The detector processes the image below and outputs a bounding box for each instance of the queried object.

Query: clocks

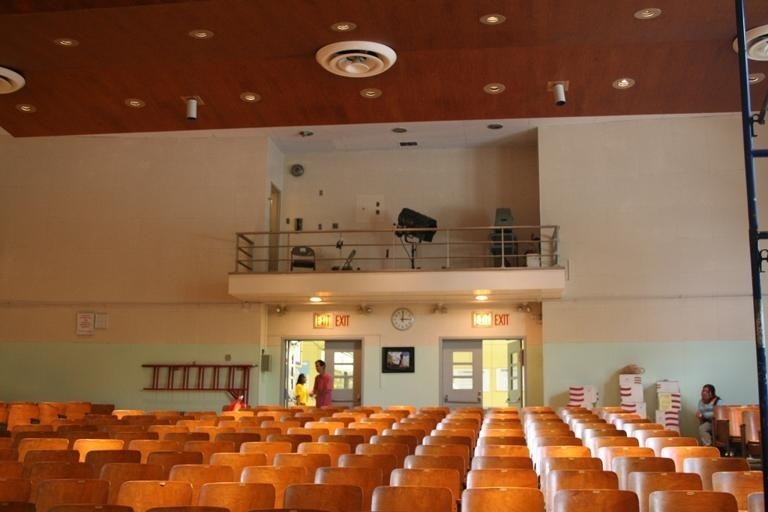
[390,306,415,330]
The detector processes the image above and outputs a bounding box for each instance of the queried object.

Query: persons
[696,384,726,456]
[293,374,307,406]
[309,360,333,408]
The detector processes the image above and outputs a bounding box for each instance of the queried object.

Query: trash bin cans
[527,253,540,267]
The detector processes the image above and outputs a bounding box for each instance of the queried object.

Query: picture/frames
[381,346,415,372]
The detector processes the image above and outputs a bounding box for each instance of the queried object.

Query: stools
[523,251,541,268]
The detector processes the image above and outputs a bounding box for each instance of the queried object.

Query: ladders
[142,364,258,390]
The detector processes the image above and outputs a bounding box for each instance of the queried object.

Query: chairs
[1,403,768,510]
[332,249,357,270]
[488,232,519,266]
[290,246,317,272]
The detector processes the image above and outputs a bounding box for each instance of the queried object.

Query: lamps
[733,24,767,65]
[185,99,197,119]
[551,83,566,107]
[313,40,397,79]
[1,66,24,96]
